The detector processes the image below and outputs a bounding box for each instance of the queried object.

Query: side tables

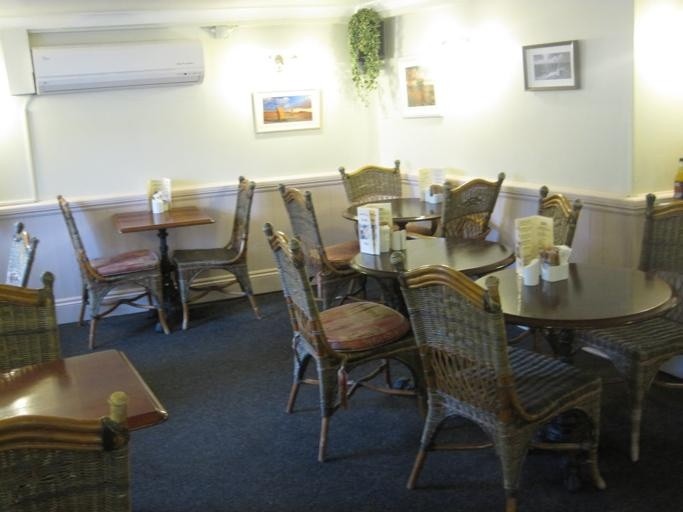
[111,205,214,331]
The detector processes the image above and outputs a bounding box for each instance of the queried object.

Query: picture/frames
[251,90,321,134]
[521,40,582,92]
[398,57,446,119]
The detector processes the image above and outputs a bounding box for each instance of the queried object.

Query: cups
[151,198,163,213]
[378,224,390,254]
[521,256,540,288]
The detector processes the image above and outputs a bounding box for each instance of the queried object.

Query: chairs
[9,223,38,286]
[0,391,129,512]
[57,195,171,350]
[0,272,60,361]
[172,176,262,331]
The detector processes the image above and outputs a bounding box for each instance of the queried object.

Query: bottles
[670,157,682,201]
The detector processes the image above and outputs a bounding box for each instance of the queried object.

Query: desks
[0,349,169,432]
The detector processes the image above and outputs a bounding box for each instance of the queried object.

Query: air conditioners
[30,39,205,96]
